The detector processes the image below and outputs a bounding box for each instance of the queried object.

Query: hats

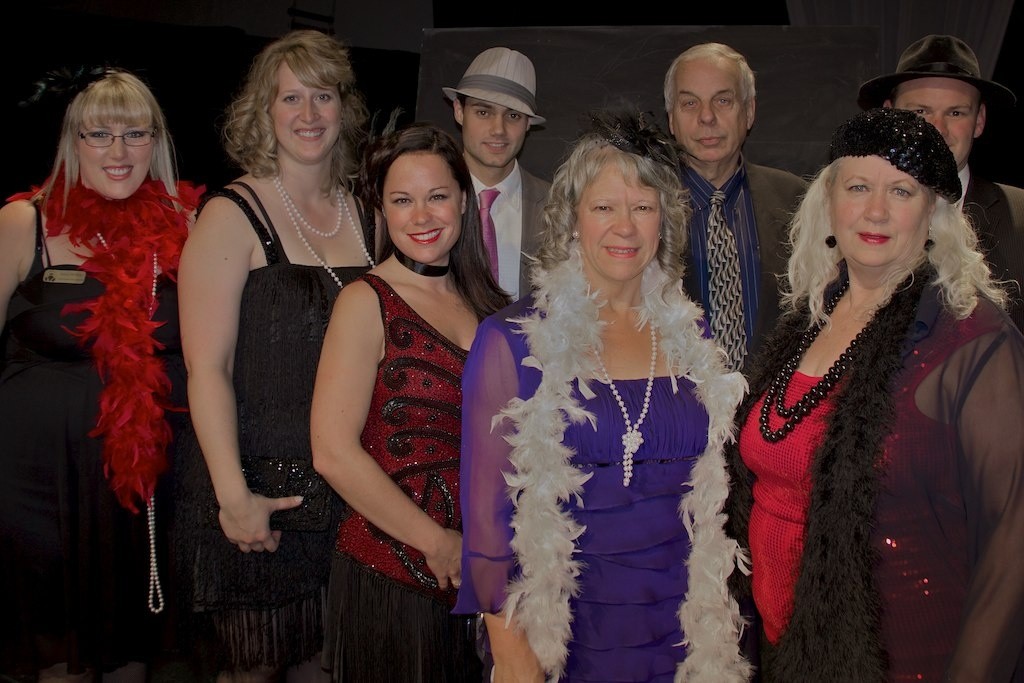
[442,47,548,125]
[856,35,1017,129]
[828,107,963,205]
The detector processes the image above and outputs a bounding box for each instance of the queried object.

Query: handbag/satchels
[207,457,333,534]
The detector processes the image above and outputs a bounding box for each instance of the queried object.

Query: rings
[452,579,463,587]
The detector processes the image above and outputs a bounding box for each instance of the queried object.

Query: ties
[477,189,500,285]
[706,191,749,372]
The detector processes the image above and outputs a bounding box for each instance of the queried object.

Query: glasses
[76,126,165,148]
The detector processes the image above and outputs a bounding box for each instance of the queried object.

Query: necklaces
[759,279,880,444]
[89,230,165,613]
[391,244,452,276]
[274,176,376,289]
[588,314,657,486]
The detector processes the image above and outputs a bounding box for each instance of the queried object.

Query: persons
[451,98,754,683]
[664,42,811,376]
[177,28,384,683]
[859,36,1024,331]
[723,106,1024,683]
[310,106,514,683]
[0,48,206,683]
[442,46,553,304]
